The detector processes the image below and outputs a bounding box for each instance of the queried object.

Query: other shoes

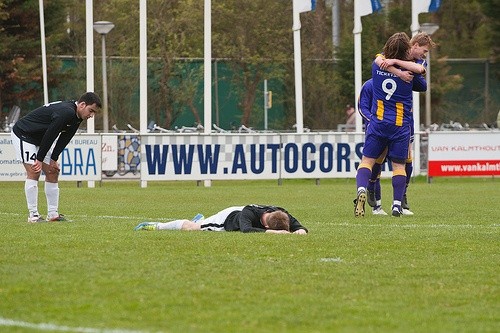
[401,208,414,215]
[353,189,367,218]
[27,215,47,222]
[46,213,68,221]
[134,221,159,231]
[391,200,402,217]
[371,206,388,216]
[191,213,205,222]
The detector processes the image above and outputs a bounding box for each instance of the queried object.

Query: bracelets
[50,159,56,163]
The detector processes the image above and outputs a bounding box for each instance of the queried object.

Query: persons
[353,32,441,218]
[11,92,102,222]
[343,104,356,132]
[134,204,308,236]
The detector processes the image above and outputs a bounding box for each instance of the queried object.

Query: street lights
[420,22,439,130]
[94,20,115,134]
